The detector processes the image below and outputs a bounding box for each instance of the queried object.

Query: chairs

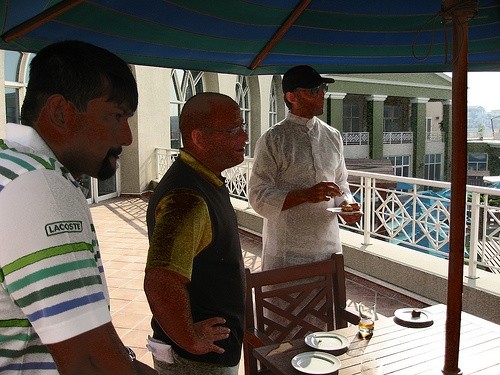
[243,252,361,375]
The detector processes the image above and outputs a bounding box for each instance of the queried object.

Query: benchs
[345,158,397,240]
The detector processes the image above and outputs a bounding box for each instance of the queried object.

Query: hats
[282,65,335,89]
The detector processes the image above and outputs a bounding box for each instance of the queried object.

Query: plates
[327,207,364,215]
[305,331,349,351]
[394,308,435,323]
[291,351,342,375]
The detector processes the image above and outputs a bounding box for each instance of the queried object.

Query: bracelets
[125,346,136,363]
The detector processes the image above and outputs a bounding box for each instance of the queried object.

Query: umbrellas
[0,0,500,375]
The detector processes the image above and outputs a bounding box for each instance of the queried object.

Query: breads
[342,203,361,211]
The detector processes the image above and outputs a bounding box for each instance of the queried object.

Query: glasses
[199,123,245,136]
[294,86,329,95]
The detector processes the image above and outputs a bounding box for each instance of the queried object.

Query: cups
[359,301,376,339]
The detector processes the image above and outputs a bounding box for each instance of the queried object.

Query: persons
[247,64,363,343]
[0,41,159,375]
[144,91,250,375]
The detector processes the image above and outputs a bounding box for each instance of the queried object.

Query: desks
[253,303,500,375]
